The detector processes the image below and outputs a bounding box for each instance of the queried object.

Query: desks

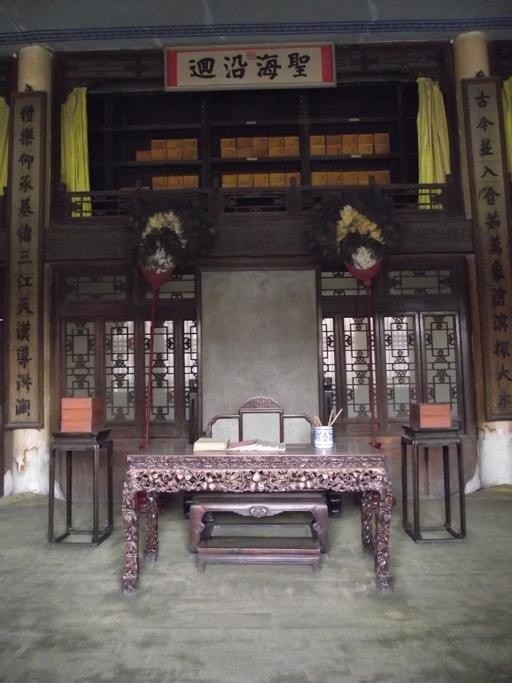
[48,428,114,549]
[123,444,394,595]
[401,425,466,545]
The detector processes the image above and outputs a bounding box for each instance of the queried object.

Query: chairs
[189,396,330,573]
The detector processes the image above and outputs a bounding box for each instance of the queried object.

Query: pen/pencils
[328,408,344,426]
[312,415,322,427]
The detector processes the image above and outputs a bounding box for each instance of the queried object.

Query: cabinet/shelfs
[87,82,416,192]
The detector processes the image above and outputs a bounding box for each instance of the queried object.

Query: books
[192,436,286,451]
